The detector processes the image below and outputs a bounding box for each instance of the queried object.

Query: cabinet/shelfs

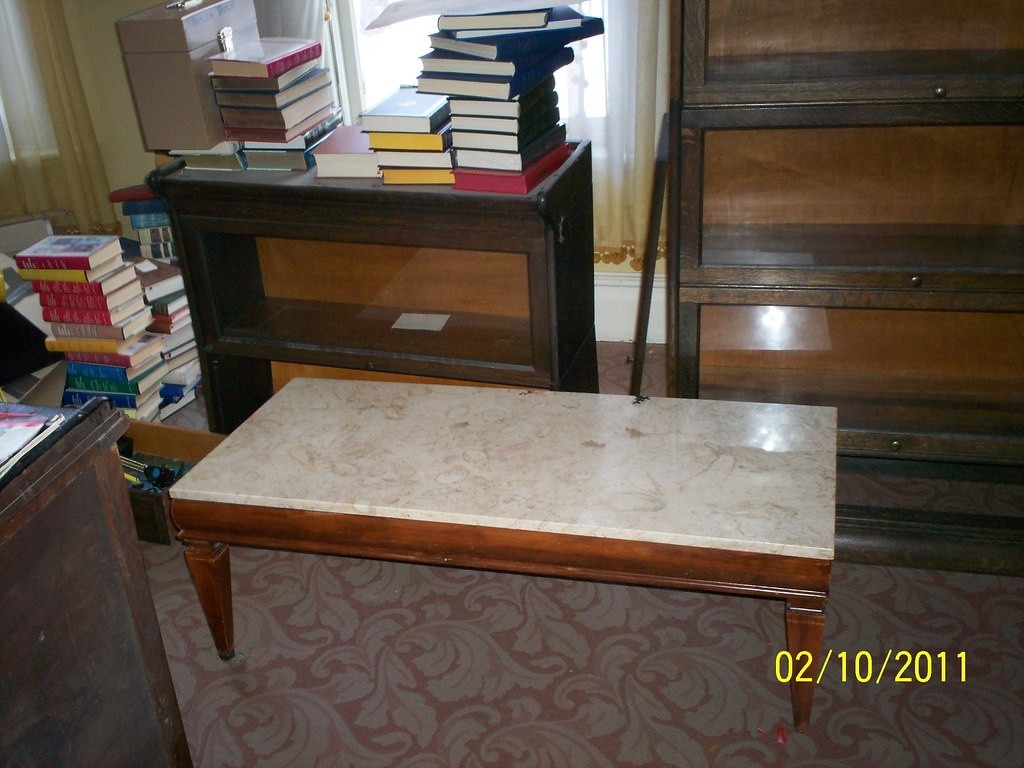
[144,140,602,433]
[664,1,1022,578]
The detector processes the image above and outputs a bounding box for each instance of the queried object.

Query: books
[0,1,607,485]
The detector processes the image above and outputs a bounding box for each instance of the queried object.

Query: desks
[170,375,841,733]
[0,396,197,768]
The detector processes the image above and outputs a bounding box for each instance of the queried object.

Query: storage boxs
[115,0,268,150]
